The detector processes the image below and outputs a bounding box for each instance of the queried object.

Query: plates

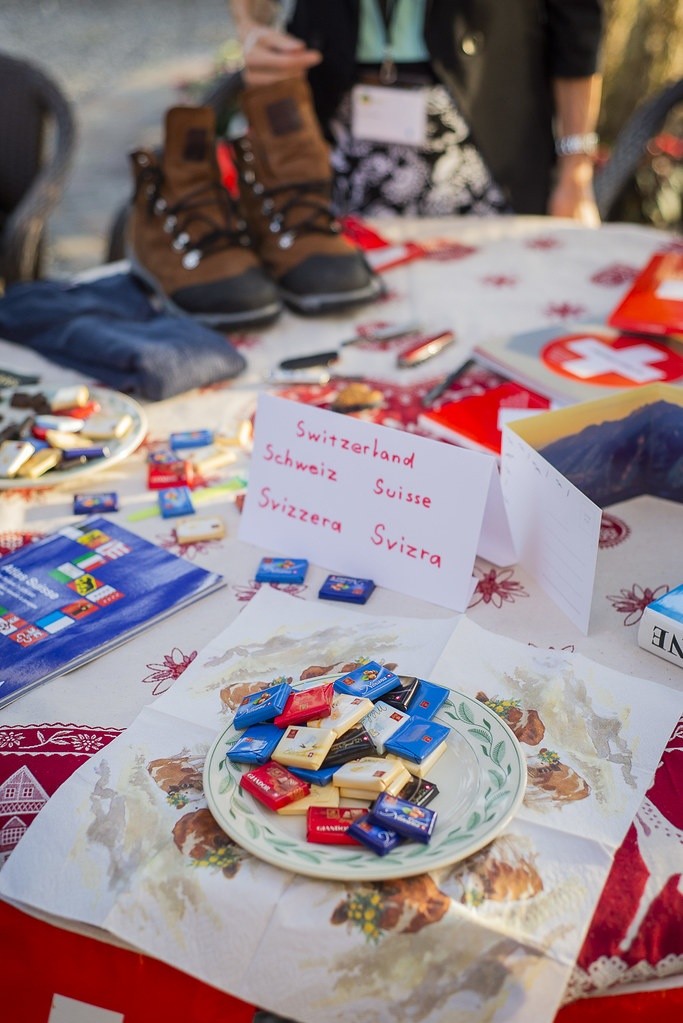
[0,386,147,491]
[205,674,525,881]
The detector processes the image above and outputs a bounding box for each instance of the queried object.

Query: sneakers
[231,77,379,312]
[122,105,282,329]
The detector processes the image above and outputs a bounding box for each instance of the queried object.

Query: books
[609,246,683,340]
[639,584,683,669]
[419,381,563,457]
[0,516,229,710]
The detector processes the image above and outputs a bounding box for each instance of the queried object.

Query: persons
[228,0,612,221]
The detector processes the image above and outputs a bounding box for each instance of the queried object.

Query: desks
[0,213,683,1023]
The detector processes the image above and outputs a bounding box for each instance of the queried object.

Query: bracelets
[556,132,599,156]
[243,30,265,53]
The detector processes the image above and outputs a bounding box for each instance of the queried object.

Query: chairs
[1,55,76,286]
